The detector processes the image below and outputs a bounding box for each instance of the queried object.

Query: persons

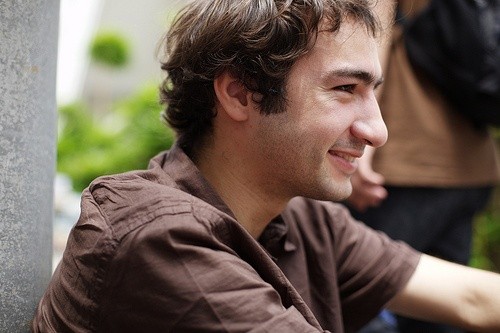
[336,0,500,333]
[26,0,500,333]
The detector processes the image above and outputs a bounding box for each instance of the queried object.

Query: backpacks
[396,0,500,133]
